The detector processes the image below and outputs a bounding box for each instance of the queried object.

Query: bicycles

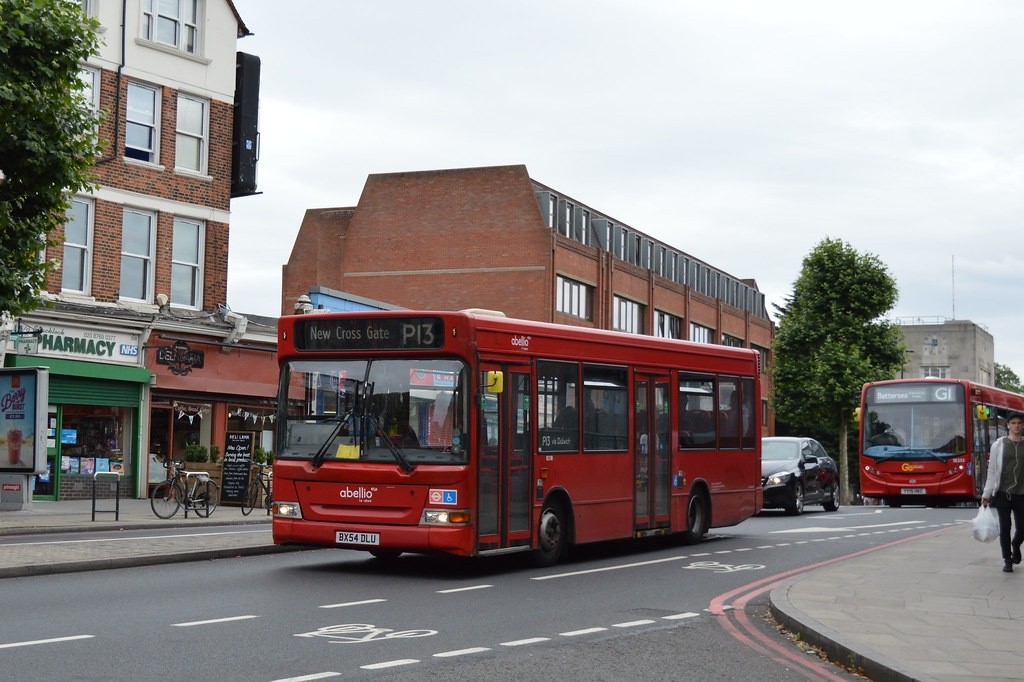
[242,464,272,516]
[151,459,220,519]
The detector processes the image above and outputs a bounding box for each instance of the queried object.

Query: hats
[1006,411,1024,423]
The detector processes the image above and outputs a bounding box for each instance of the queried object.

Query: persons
[488,437,497,447]
[981,412,1024,572]
[729,391,748,437]
[884,417,906,445]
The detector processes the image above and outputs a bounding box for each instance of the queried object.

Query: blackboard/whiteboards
[217,431,256,507]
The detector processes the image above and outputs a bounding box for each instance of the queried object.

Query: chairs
[586,408,731,450]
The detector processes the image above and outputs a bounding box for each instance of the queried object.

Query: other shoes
[1002,559,1013,572]
[1012,544,1022,565]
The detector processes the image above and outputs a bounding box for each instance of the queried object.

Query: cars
[761,436,840,515]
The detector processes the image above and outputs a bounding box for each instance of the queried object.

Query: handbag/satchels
[971,503,1000,543]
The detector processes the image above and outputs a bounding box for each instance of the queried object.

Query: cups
[8,431,23,464]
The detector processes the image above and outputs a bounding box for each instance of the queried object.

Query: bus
[271,309,764,567]
[853,376,1024,508]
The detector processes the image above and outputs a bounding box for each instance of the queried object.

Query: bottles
[393,418,397,433]
